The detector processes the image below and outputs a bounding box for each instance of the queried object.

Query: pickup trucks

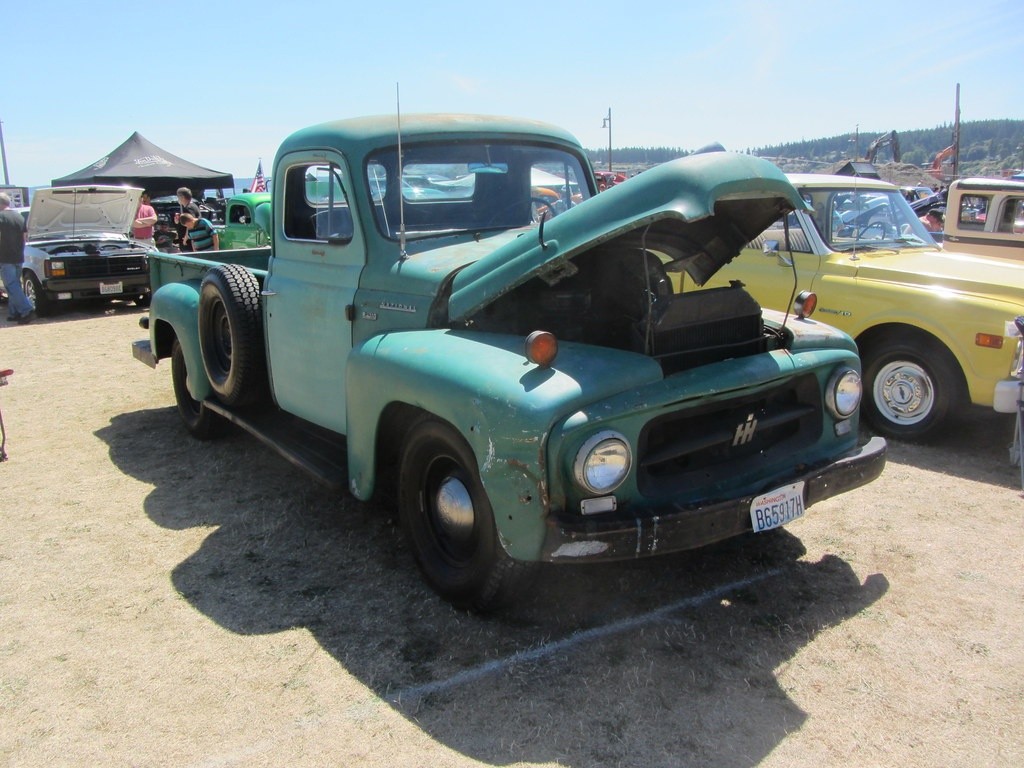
[133,114,886,617]
[937,178,1024,261]
[210,193,271,249]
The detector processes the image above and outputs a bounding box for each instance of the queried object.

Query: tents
[433,162,578,188]
[51,131,235,200]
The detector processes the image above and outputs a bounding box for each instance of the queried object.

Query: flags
[251,161,265,192]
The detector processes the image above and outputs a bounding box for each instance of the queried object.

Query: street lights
[600,107,612,172]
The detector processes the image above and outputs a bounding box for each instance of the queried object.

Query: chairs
[472,171,526,226]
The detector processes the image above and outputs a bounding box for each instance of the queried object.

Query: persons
[916,180,923,187]
[131,195,157,248]
[551,185,577,218]
[931,183,950,202]
[174,187,200,253]
[903,209,944,235]
[0,193,37,325]
[832,201,844,232]
[153,223,182,253]
[180,213,219,252]
[599,175,616,193]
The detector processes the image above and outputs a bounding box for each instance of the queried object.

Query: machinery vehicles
[924,145,955,178]
[865,130,900,164]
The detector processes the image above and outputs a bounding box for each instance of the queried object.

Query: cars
[0,185,160,317]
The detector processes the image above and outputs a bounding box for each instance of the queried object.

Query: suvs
[639,173,1024,440]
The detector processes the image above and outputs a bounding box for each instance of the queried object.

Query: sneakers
[6,310,38,325]
